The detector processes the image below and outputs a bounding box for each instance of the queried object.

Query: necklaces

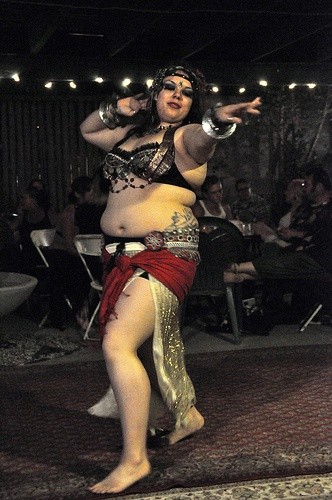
[141,124,174,136]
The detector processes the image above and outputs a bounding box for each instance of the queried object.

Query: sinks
[0,272,39,317]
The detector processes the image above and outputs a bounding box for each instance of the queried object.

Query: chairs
[73,234,104,340]
[183,216,247,345]
[31,230,72,330]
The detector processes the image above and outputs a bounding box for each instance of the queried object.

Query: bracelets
[202,105,237,140]
[99,92,122,129]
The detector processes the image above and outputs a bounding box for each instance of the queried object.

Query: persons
[221,175,332,283]
[45,175,94,332]
[79,62,261,495]
[8,179,55,275]
[277,178,311,230]
[230,180,270,225]
[190,174,233,221]
[75,165,110,232]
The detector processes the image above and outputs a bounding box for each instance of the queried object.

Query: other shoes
[217,312,233,333]
[239,308,272,336]
[279,301,304,324]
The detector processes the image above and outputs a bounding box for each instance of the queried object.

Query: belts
[104,242,198,254]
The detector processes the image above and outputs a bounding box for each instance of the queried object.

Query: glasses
[238,187,249,191]
